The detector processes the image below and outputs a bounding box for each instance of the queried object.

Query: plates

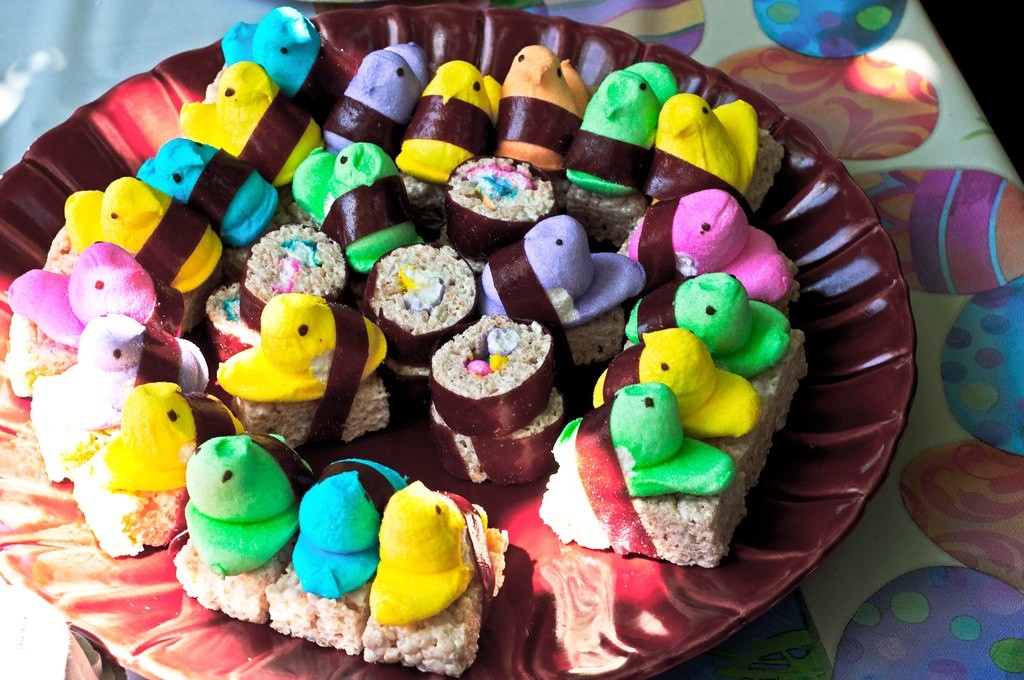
[0,3,918,680]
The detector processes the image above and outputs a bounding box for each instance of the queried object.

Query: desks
[0,0,1024,680]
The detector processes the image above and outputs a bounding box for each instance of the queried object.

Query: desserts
[0,6,810,673]
